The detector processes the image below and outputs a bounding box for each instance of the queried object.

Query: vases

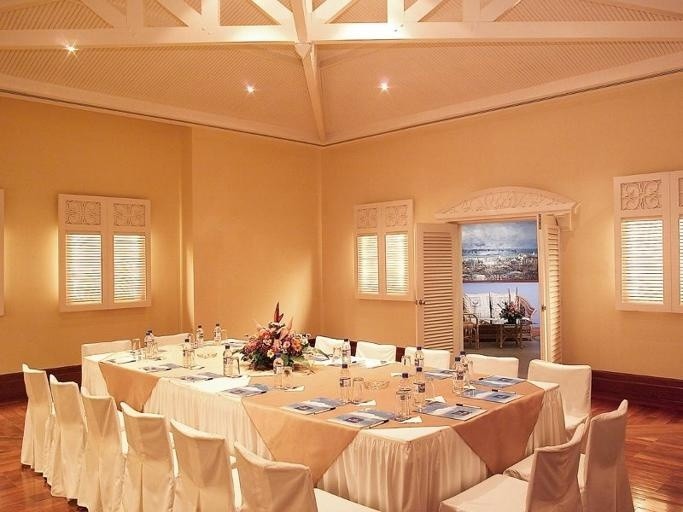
[265,358,295,370]
[507,319,518,325]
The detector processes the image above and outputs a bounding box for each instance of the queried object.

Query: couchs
[463,292,538,342]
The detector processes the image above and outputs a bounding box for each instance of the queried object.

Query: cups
[231,357,240,377]
[331,346,342,367]
[221,329,227,344]
[352,377,366,404]
[282,368,294,392]
[400,355,412,378]
[393,390,412,421]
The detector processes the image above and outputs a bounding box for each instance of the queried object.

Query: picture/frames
[463,247,539,285]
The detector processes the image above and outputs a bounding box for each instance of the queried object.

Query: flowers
[232,302,328,376]
[498,286,526,320]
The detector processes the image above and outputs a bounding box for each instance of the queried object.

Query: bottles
[413,368,425,404]
[273,353,284,388]
[223,345,233,375]
[400,373,413,415]
[144,333,152,359]
[339,364,352,403]
[342,338,351,366]
[414,347,424,368]
[459,351,468,387]
[214,324,222,344]
[148,331,155,358]
[183,339,191,365]
[451,357,464,392]
[196,326,204,347]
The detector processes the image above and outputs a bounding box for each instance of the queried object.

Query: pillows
[463,291,490,318]
[490,291,516,318]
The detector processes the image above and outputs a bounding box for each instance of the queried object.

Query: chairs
[405,346,451,372]
[529,359,592,443]
[154,332,191,347]
[48,374,124,500]
[172,418,243,512]
[77,386,176,512]
[463,313,480,350]
[355,340,397,363]
[120,400,236,512]
[20,363,57,477]
[466,354,519,378]
[315,336,349,355]
[438,421,587,512]
[234,441,383,512]
[502,398,635,512]
[81,340,132,366]
